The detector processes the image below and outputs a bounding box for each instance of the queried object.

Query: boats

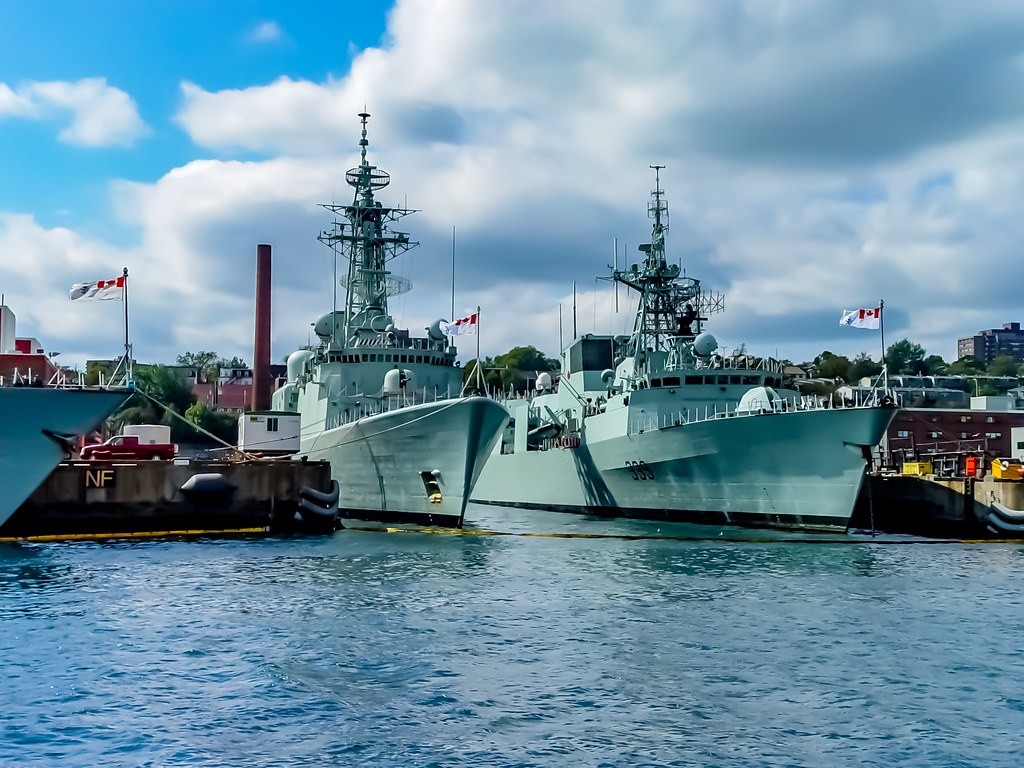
[848,299,1024,540]
[0,266,136,528]
[272,104,512,530]
[0,242,340,541]
[469,162,899,534]
[527,423,563,445]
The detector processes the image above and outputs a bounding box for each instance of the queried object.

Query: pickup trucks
[80,435,176,461]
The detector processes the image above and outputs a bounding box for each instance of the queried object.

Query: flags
[442,313,477,337]
[839,308,880,329]
[68,275,125,301]
